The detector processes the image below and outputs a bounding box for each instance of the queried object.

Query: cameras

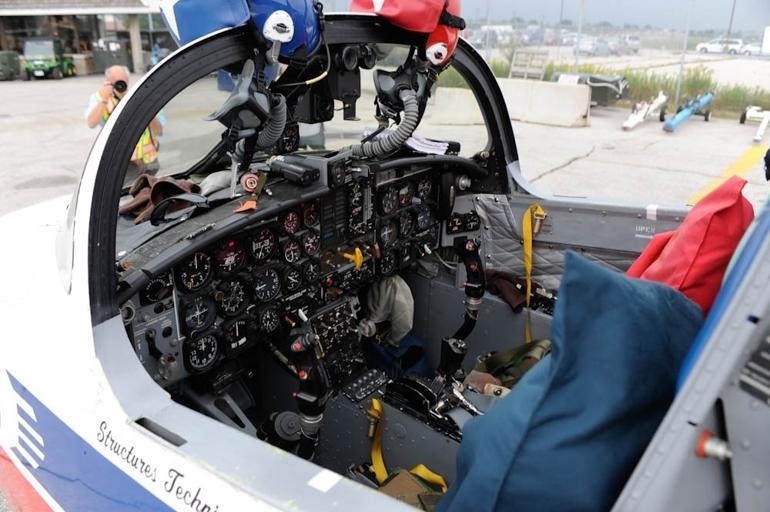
[111,80,127,99]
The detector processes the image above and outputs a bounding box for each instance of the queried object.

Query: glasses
[150,193,210,226]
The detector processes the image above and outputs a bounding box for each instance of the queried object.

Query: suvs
[20,37,76,80]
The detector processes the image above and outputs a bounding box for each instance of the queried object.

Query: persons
[87,65,165,189]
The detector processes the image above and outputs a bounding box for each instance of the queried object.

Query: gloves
[119,174,203,225]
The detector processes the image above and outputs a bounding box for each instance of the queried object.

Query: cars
[695,36,762,57]
[466,25,640,56]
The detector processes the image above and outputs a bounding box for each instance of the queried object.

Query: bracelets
[99,97,108,104]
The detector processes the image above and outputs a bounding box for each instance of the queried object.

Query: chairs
[432,180,769,511]
